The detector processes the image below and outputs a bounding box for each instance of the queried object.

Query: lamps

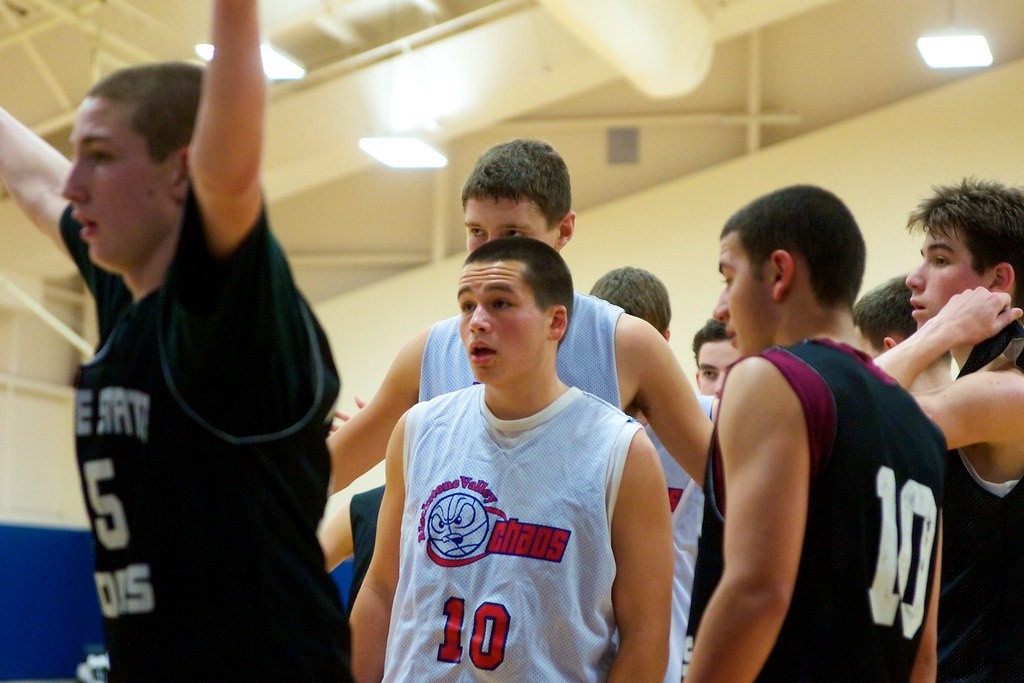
[916,0,994,69]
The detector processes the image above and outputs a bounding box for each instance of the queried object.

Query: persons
[326,139,740,683]
[874,179,1023,683]
[681,184,947,683]
[348,238,673,683]
[855,273,952,395]
[1,1,354,683]
